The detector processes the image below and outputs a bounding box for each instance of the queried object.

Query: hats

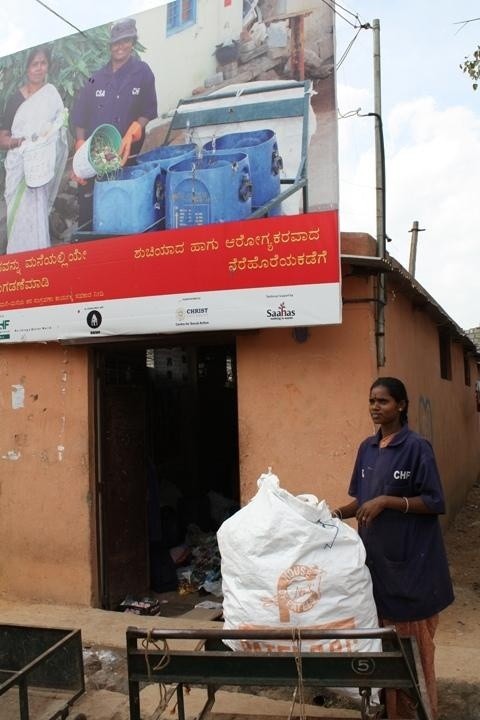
[110,22,137,43]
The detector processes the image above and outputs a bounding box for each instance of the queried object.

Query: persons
[1,47,68,254]
[331,376,456,720]
[73,18,158,230]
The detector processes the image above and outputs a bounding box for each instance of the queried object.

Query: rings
[362,516,366,521]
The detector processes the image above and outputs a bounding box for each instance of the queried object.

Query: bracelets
[331,510,339,517]
[401,497,409,514]
[335,508,342,521]
